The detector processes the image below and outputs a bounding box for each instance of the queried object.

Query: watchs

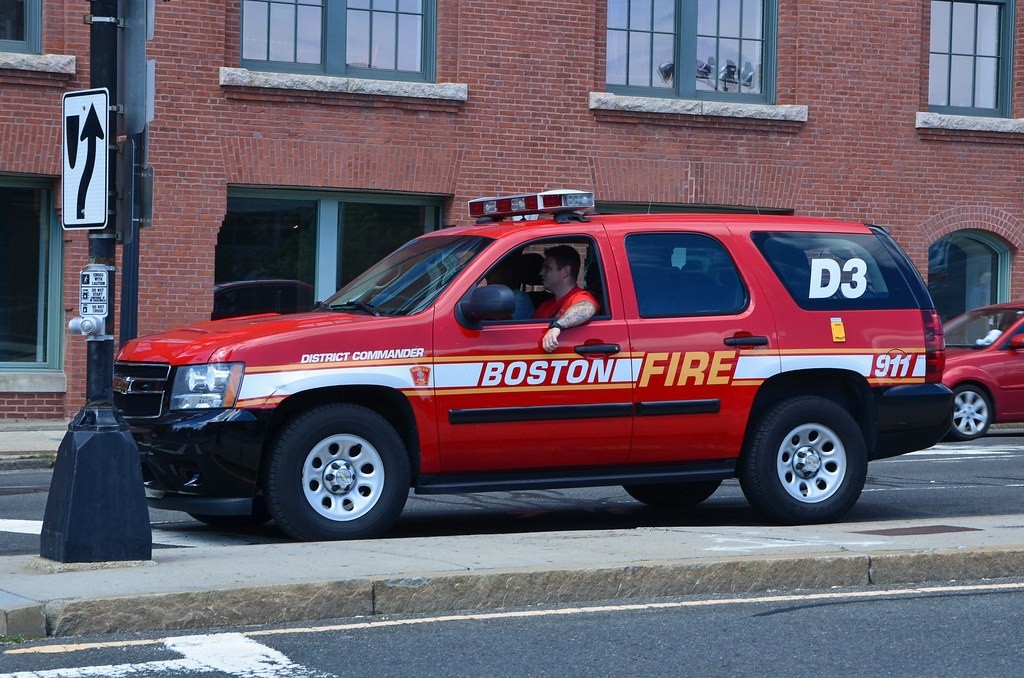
[548,321,562,331]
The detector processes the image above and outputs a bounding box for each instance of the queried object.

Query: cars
[944,301,1024,440]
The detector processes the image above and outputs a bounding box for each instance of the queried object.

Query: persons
[484,245,600,353]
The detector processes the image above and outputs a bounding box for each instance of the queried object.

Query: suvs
[108,186,956,539]
